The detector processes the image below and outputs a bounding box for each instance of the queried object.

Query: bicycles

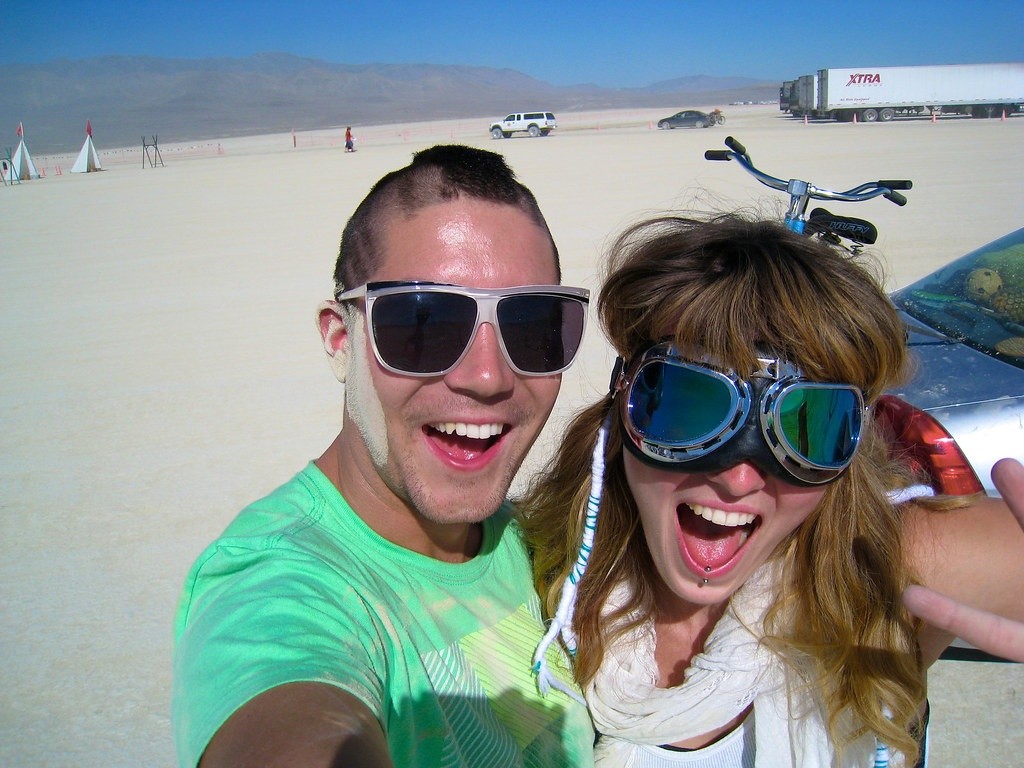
[708,111,726,126]
[710,139,912,258]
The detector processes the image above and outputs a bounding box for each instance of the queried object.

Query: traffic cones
[802,114,808,126]
[1000,109,1007,123]
[853,113,857,124]
[932,113,936,123]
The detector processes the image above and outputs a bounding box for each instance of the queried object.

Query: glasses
[608,343,870,486]
[332,279,590,379]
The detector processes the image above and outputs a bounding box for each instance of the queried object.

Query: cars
[876,231,1024,496]
[657,110,717,130]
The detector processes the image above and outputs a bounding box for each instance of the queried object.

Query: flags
[87,121,92,136]
[16,123,24,137]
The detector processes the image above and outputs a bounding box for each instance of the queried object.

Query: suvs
[488,111,557,140]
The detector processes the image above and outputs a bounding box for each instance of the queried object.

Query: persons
[173,140,596,768]
[517,213,1024,768]
[345,127,353,152]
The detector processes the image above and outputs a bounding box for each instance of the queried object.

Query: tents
[4,140,38,182]
[71,135,102,172]
